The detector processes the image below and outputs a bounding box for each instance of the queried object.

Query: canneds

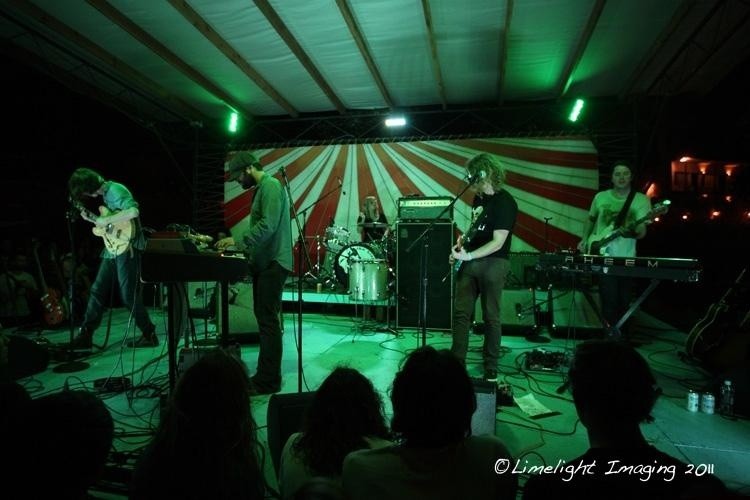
[316,283,323,293]
[687,389,699,412]
[701,392,716,414]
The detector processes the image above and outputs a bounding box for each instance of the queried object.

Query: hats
[227,150,256,182]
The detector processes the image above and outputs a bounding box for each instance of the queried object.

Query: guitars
[32,247,65,326]
[686,268,749,359]
[69,195,135,255]
[578,203,669,254]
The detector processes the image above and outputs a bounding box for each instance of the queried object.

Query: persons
[447,153,518,381]
[57,168,159,348]
[0,250,40,330]
[140,337,728,500]
[574,158,653,336]
[357,196,388,321]
[213,150,295,396]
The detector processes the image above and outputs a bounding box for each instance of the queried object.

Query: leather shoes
[486,368,498,379]
[61,337,88,350]
[128,336,158,347]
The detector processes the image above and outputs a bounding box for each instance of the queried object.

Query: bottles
[719,380,736,421]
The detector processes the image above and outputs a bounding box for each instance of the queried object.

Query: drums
[349,259,389,301]
[324,226,351,249]
[333,241,386,289]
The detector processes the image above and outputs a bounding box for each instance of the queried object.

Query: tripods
[301,238,337,289]
[377,250,403,335]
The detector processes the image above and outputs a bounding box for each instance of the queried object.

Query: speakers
[163,278,218,318]
[217,282,284,345]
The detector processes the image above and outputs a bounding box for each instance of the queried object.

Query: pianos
[552,252,700,270]
[141,235,247,283]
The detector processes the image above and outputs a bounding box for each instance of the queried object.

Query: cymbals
[359,222,387,228]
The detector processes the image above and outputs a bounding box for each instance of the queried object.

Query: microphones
[281,166,288,178]
[470,170,486,184]
[337,178,347,197]
